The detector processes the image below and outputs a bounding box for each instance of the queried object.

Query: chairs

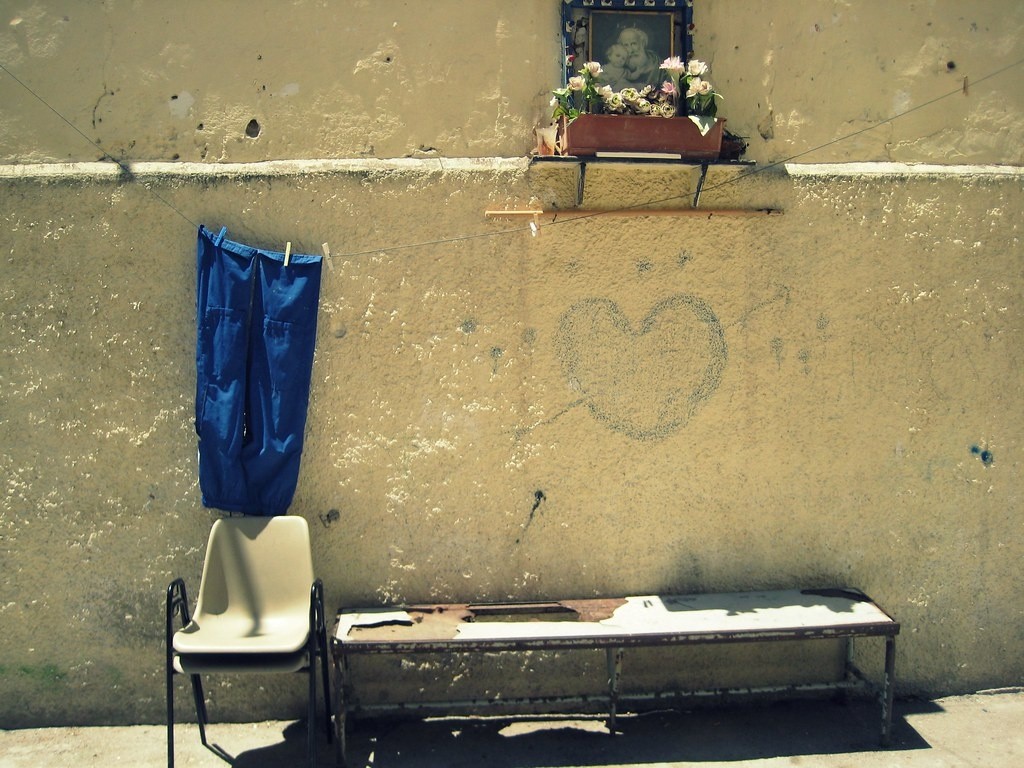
[166,515,332,768]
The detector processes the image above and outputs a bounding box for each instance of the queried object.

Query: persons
[598,27,667,94]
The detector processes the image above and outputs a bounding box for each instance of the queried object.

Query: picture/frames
[562,0,692,110]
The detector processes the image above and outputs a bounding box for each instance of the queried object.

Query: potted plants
[550,56,726,161]
